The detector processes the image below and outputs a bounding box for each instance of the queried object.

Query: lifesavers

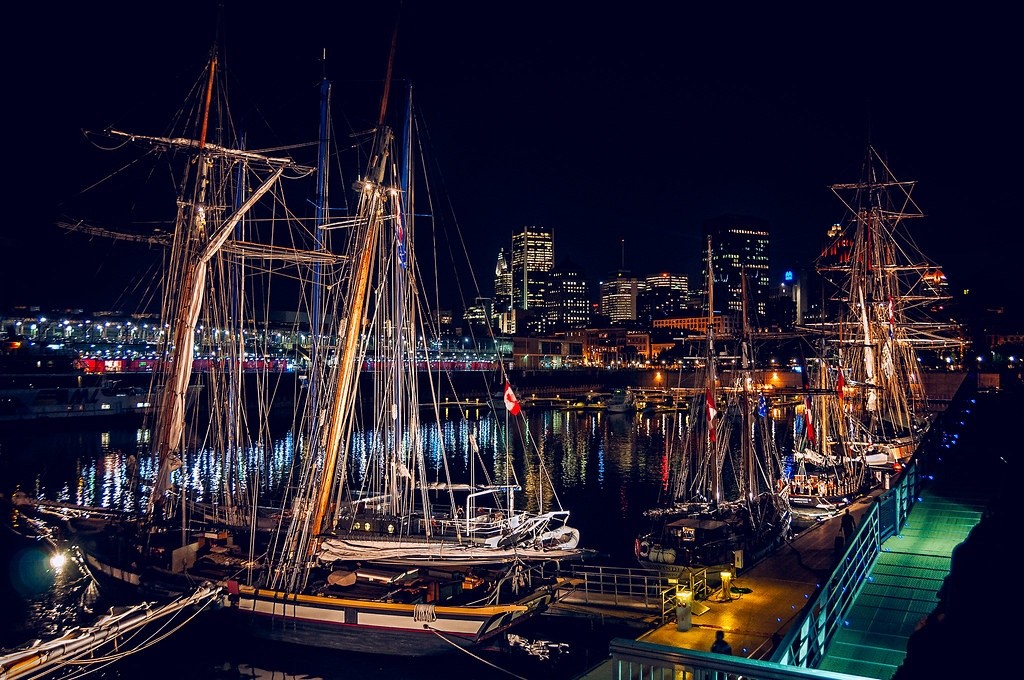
[640,541,650,558]
[634,539,640,555]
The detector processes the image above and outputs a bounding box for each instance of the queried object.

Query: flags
[503,379,521,415]
[805,398,816,446]
[838,365,846,399]
[887,293,894,324]
[753,393,769,422]
[706,387,718,442]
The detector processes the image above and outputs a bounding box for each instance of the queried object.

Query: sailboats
[625,142,973,570]
[6,53,587,655]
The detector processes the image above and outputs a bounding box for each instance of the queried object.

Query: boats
[2,371,204,421]
[558,387,801,414]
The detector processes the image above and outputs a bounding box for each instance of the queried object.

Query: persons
[711,630,732,680]
[377,511,450,537]
[457,505,464,518]
[839,509,856,546]
[549,575,558,602]
[475,506,484,517]
